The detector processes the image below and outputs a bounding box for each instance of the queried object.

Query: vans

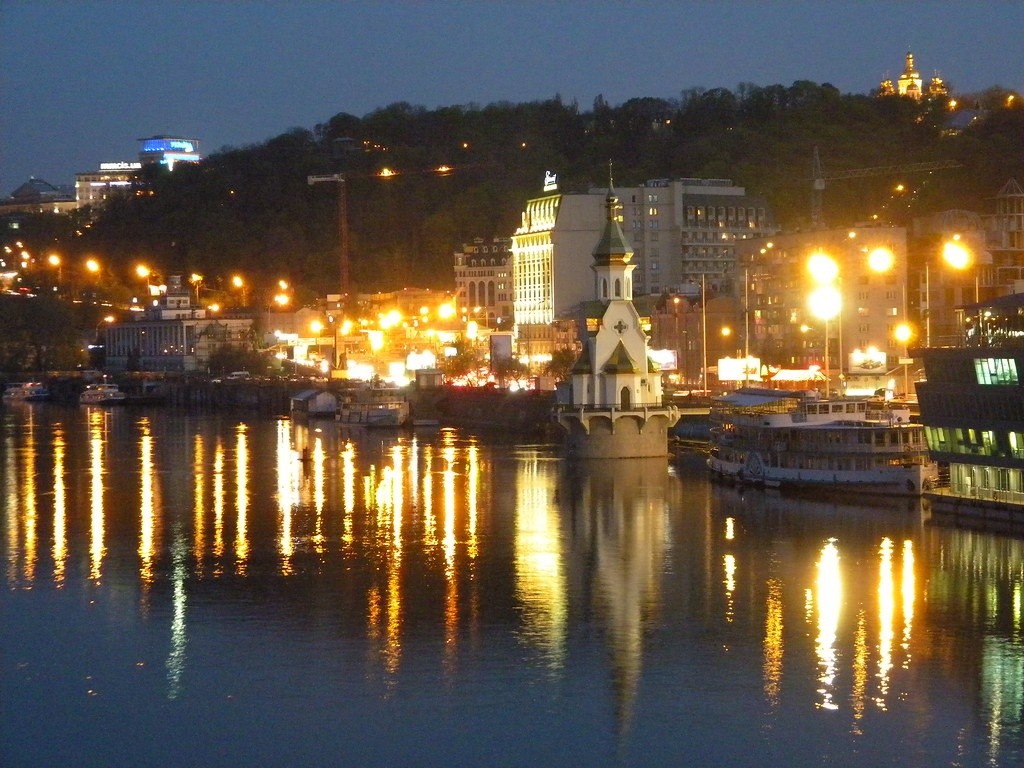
[227,371,250,381]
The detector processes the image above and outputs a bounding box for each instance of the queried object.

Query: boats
[79,383,125,405]
[909,293,1024,539]
[706,387,938,496]
[335,380,409,426]
[3,382,51,401]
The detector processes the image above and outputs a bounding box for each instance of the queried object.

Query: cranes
[307,165,460,304]
